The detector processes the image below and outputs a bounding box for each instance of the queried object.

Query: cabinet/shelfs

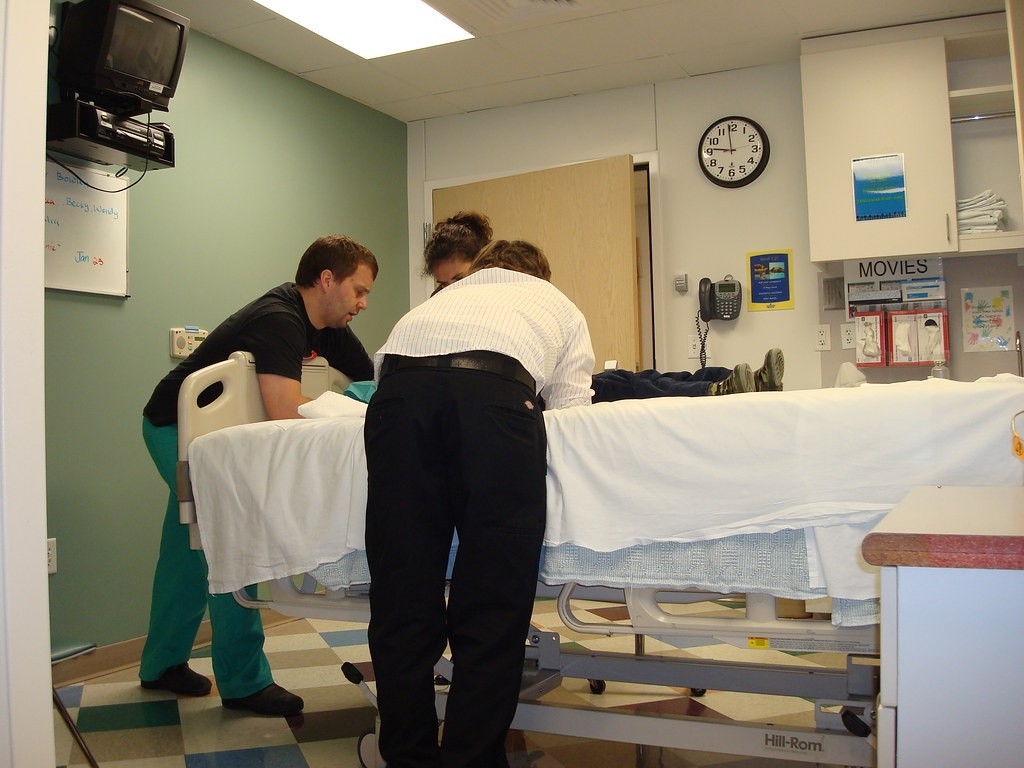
[800,0,1024,272]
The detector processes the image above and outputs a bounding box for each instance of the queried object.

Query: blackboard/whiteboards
[45,150,132,299]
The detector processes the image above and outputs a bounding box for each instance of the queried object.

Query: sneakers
[708,364,756,395]
[752,348,785,391]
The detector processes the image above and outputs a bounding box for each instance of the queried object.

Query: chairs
[51,641,99,768]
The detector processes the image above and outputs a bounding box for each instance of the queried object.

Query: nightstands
[862,485,1024,768]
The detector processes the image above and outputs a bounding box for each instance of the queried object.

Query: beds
[178,352,1023,768]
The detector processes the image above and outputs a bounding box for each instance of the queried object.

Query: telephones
[699,274,743,322]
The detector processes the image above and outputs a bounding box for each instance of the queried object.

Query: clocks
[698,116,770,188]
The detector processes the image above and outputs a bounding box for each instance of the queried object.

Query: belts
[379,350,536,393]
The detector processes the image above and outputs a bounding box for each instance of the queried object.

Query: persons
[362,238,591,768]
[592,348,783,403]
[142,235,378,712]
[419,210,494,297]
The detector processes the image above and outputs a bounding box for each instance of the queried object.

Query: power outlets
[47,538,57,575]
[841,323,856,349]
[815,324,831,350]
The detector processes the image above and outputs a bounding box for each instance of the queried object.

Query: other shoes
[141,663,212,697]
[221,683,304,716]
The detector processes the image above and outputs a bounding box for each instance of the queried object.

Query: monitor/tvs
[56,0,190,113]
